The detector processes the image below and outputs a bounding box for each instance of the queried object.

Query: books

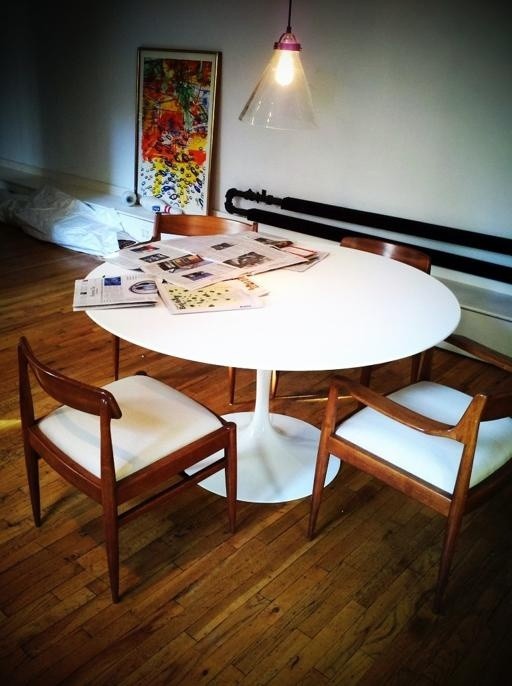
[71,229,331,316]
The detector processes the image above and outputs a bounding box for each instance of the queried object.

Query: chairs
[305,329,510,614]
[16,333,243,603]
[113,210,255,408]
[270,233,433,411]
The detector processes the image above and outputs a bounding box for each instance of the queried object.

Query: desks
[79,233,466,506]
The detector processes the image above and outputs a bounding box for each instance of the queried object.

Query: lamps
[234,0,321,134]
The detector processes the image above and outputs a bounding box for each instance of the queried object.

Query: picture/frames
[133,44,225,219]
